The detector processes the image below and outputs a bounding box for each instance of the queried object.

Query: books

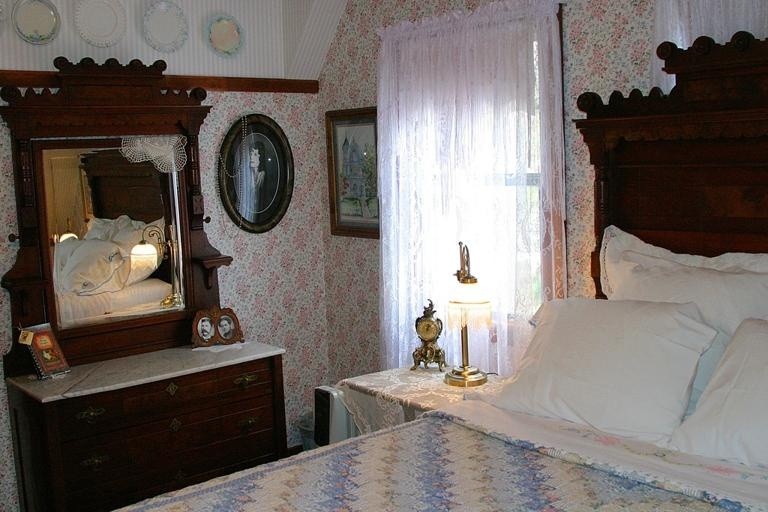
[17,322,73,378]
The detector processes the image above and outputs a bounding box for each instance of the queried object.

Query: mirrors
[1,57,232,378]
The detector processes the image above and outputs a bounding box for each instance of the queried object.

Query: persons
[217,319,234,338]
[248,144,271,202]
[199,319,214,338]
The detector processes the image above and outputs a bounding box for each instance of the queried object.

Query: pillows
[126,242,162,287]
[62,239,131,296]
[85,218,114,242]
[597,221,768,338]
[113,223,145,250]
[495,295,705,440]
[674,316,768,464]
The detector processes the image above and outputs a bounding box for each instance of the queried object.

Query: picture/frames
[325,106,379,240]
[219,112,297,233]
[189,306,246,349]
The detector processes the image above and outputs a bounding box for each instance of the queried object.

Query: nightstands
[336,360,497,441]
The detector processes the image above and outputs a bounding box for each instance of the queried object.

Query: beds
[56,151,171,325]
[117,30,768,510]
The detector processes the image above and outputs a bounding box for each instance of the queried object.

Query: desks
[4,334,286,511]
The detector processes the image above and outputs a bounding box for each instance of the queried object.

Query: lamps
[439,242,495,388]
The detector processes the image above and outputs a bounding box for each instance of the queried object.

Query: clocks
[402,297,450,371]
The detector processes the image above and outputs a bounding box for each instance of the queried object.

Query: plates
[141,0,189,54]
[73,1,128,49]
[206,13,245,56]
[10,1,60,46]
